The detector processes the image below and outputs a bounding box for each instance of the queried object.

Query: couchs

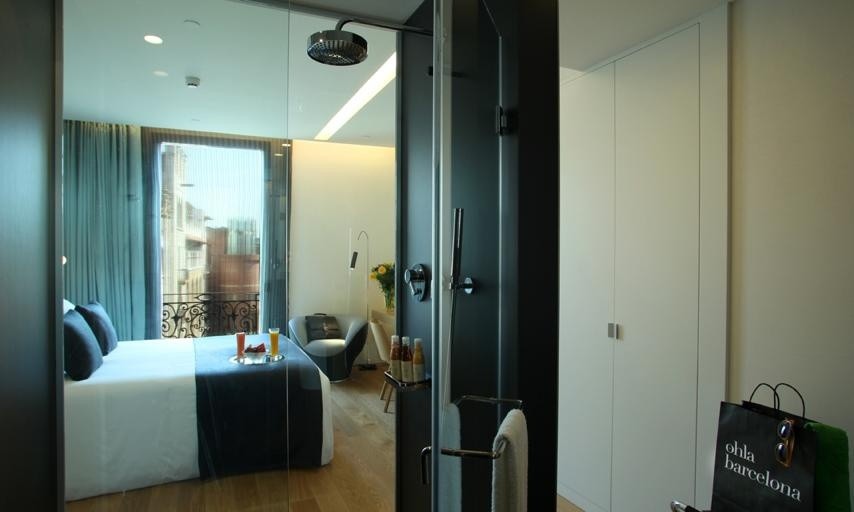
[286,311,368,385]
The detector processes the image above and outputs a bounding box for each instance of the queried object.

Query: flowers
[370,263,399,314]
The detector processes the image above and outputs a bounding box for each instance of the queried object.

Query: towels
[61,306,105,381]
[75,301,121,358]
[489,407,532,512]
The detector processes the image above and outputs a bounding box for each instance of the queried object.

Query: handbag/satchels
[710,383,851,512]
[305,312,340,345]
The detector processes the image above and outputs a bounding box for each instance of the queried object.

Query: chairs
[365,314,399,410]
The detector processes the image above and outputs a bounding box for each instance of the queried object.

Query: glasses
[773,415,798,470]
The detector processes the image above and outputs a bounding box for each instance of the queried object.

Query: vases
[382,294,394,314]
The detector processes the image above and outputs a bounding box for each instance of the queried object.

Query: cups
[267,327,279,361]
[234,331,245,361]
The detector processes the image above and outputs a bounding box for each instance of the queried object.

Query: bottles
[388,333,425,384]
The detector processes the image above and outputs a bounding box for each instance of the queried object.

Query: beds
[61,289,336,502]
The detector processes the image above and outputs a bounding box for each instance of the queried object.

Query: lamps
[351,232,377,371]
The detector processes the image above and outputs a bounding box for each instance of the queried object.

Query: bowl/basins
[241,349,268,362]
